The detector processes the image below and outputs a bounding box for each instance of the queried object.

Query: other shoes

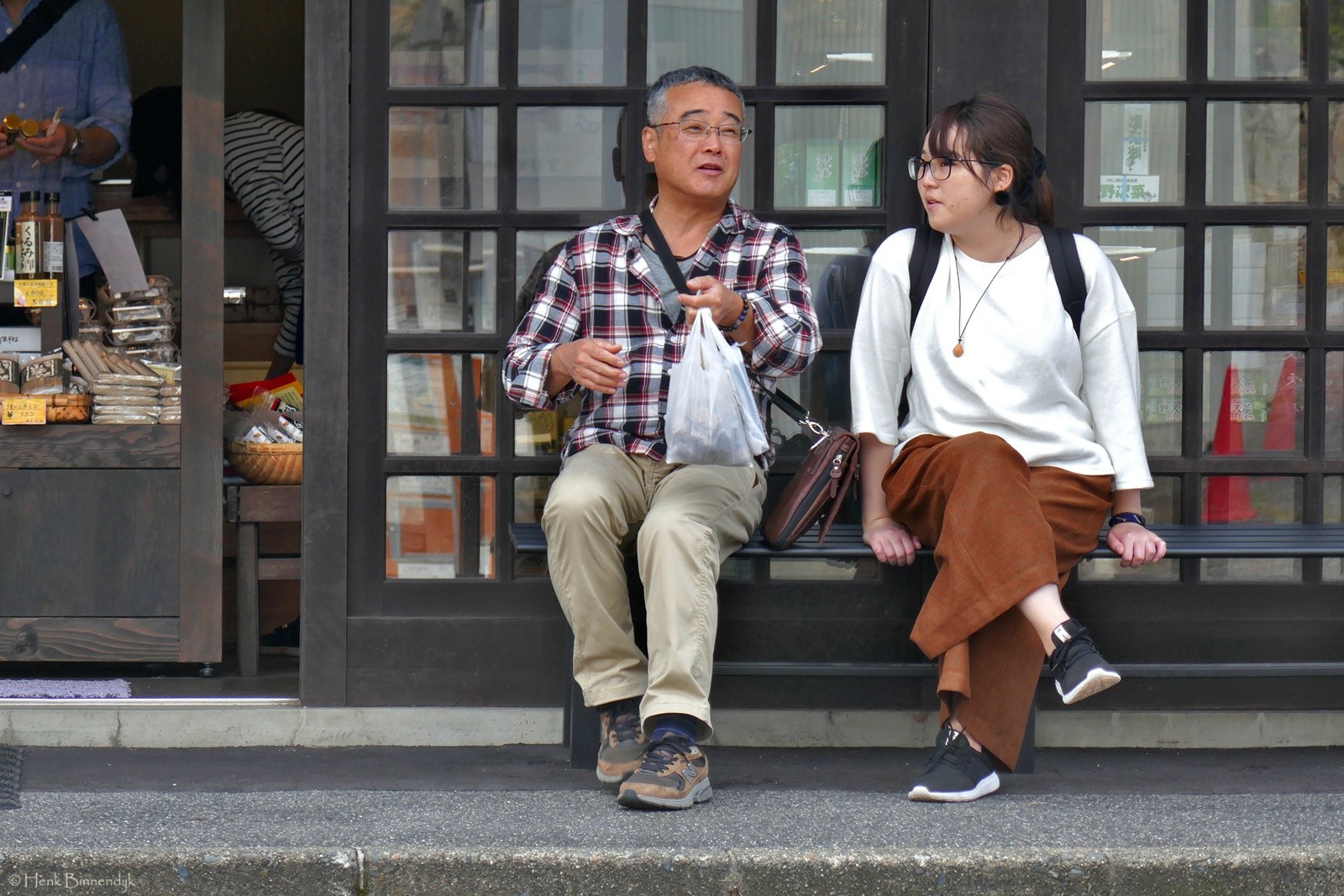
[259,625,299,658]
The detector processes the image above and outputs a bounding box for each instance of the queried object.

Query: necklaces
[642,237,699,262]
[951,217,1026,359]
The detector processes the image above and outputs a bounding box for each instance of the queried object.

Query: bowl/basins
[22,275,183,364]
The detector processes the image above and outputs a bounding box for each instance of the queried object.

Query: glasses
[650,120,753,145]
[907,157,1001,180]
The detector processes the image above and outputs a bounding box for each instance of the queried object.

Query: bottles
[0,113,23,147]
[13,118,39,150]
[12,190,41,280]
[40,192,65,282]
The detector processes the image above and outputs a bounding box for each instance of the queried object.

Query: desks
[225,482,301,677]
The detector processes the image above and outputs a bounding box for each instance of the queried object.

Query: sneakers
[1051,618,1120,704]
[617,736,713,810]
[907,723,1000,802]
[595,696,644,783]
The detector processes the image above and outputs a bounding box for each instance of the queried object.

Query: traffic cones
[1264,356,1298,451]
[1203,364,1259,524]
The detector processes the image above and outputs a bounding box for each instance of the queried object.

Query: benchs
[506,517,1344,777]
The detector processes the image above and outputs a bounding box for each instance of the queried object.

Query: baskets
[227,442,302,486]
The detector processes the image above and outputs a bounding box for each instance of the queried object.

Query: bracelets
[1108,513,1147,527]
[717,291,750,332]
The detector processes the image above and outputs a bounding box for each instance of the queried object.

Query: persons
[850,97,1167,803]
[0,0,133,327]
[500,66,823,809]
[131,86,305,381]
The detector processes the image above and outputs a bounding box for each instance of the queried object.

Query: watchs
[66,127,83,158]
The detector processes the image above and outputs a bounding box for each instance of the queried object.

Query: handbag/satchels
[761,426,860,550]
[666,307,769,467]
